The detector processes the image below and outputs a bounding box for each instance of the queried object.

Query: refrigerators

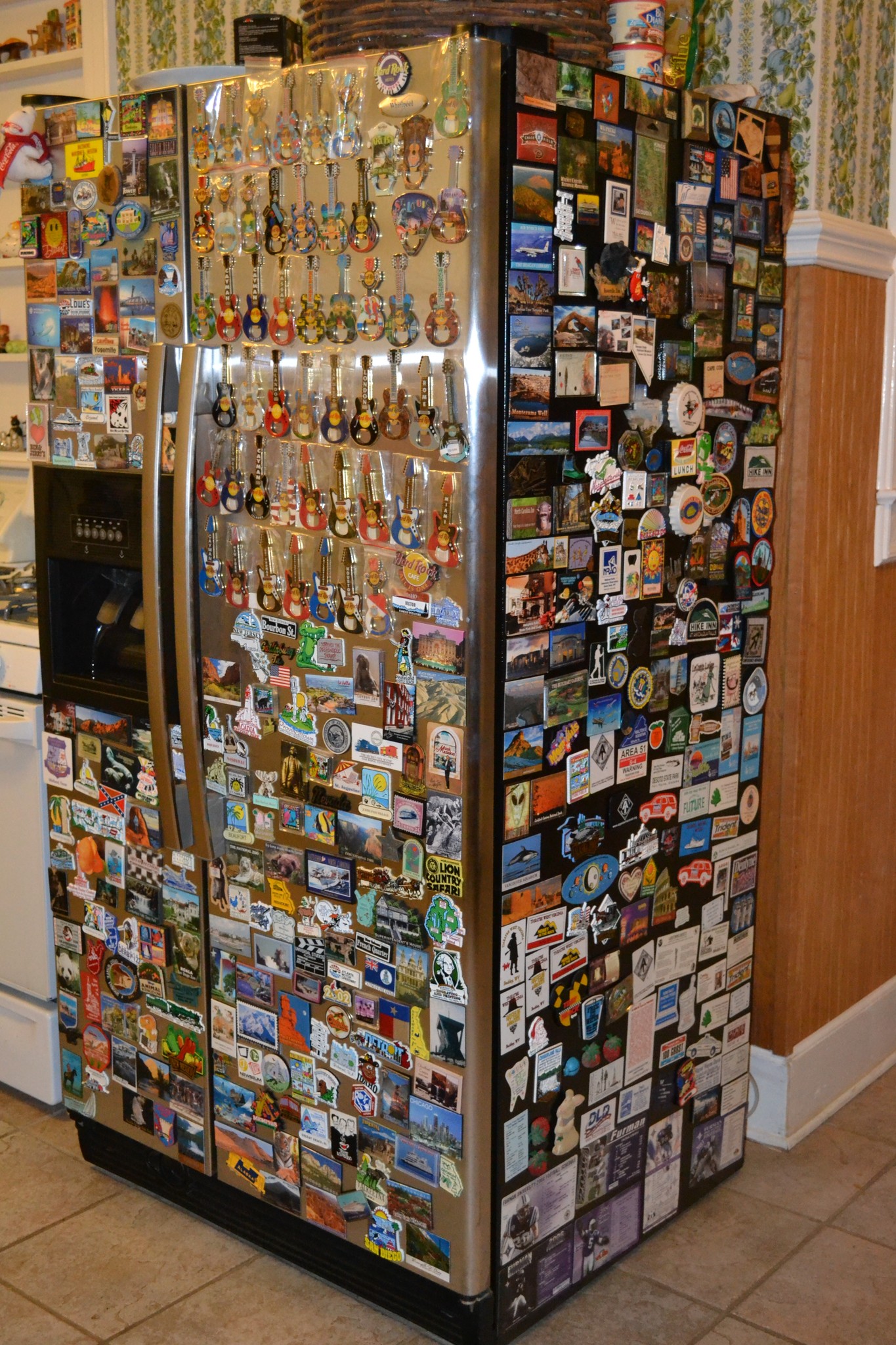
[0,19,796,1340]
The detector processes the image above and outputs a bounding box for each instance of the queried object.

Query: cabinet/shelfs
[0,2,120,629]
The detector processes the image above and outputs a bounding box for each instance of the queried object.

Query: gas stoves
[1,558,36,629]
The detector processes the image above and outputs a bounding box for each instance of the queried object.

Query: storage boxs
[233,13,303,72]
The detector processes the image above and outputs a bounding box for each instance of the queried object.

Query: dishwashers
[0,689,61,1114]
